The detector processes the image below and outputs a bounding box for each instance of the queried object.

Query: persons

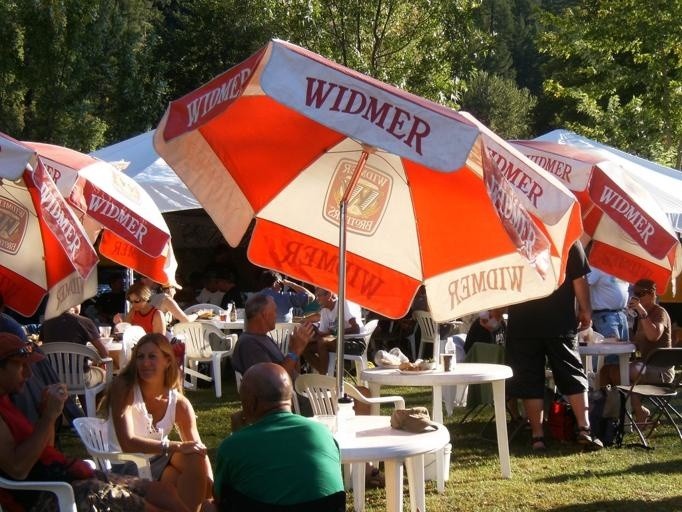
[0,271,365,512]
[365,239,682,450]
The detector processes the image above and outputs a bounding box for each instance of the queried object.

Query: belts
[592,309,617,314]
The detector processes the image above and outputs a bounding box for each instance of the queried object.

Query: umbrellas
[19,141,183,290]
[0,131,98,321]
[153,39,583,398]
[504,140,682,298]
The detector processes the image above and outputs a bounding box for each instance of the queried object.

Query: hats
[0,333,44,363]
[629,277,656,293]
[390,406,438,433]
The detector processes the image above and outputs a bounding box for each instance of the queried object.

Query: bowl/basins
[100,338,113,346]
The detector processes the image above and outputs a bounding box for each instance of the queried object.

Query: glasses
[2,342,33,358]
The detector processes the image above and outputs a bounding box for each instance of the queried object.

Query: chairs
[612,348,680,448]
[293,373,405,417]
[71,415,156,480]
[2,478,78,511]
[38,343,116,417]
[1,293,681,377]
[172,321,243,399]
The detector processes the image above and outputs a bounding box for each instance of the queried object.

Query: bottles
[445,337,456,368]
[374,347,409,369]
[227,302,236,323]
[337,393,356,438]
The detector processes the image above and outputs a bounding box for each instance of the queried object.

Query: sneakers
[635,406,650,428]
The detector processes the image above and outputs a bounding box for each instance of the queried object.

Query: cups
[219,309,226,321]
[442,355,452,371]
[99,326,111,337]
[315,415,336,433]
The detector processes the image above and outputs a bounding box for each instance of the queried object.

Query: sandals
[532,438,547,454]
[577,426,603,449]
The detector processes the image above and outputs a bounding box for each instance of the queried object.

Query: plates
[397,369,435,374]
